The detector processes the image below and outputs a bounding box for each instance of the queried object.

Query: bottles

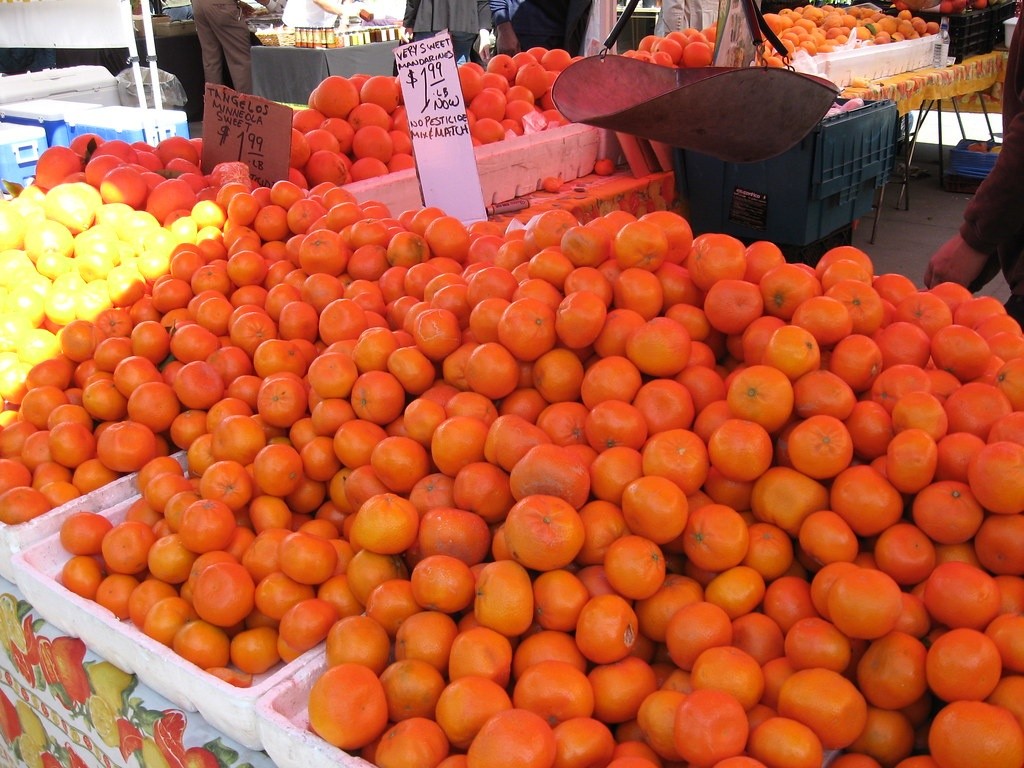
[933,17,951,68]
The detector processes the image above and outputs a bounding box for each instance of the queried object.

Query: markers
[485,199,530,215]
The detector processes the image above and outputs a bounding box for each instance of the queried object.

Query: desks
[488,167,682,223]
[850,49,1004,213]
[107,33,263,121]
[251,40,399,105]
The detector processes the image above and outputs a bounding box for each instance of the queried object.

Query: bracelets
[237,0,240,4]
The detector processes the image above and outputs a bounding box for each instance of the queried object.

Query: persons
[281,0,373,29]
[161,0,285,96]
[403,0,764,61]
[922,7,1024,336]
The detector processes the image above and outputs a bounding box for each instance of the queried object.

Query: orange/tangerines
[276,46,594,188]
[0,139,1024,768]
[618,0,989,68]
[594,158,615,175]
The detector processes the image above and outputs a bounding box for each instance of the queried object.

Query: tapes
[572,187,587,199]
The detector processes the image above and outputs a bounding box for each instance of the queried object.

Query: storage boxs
[853,0,1016,63]
[942,171,984,194]
[0,451,376,768]
[0,64,189,195]
[743,223,855,267]
[597,124,628,166]
[156,19,196,37]
[686,98,899,246]
[347,122,599,216]
[1002,16,1019,47]
[948,138,1003,178]
